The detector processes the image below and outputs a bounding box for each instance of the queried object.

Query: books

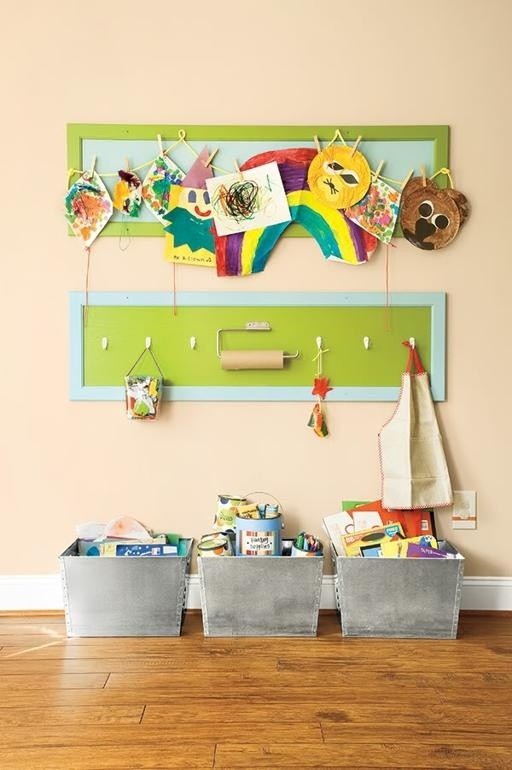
[198,538,324,637]
[332,540,466,638]
[59,537,194,638]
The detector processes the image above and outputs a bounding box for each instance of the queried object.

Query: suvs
[234,491,277,544]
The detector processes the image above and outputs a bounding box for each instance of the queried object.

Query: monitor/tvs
[321,500,456,558]
[77,533,187,556]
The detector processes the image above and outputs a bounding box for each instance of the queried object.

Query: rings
[296,533,320,552]
